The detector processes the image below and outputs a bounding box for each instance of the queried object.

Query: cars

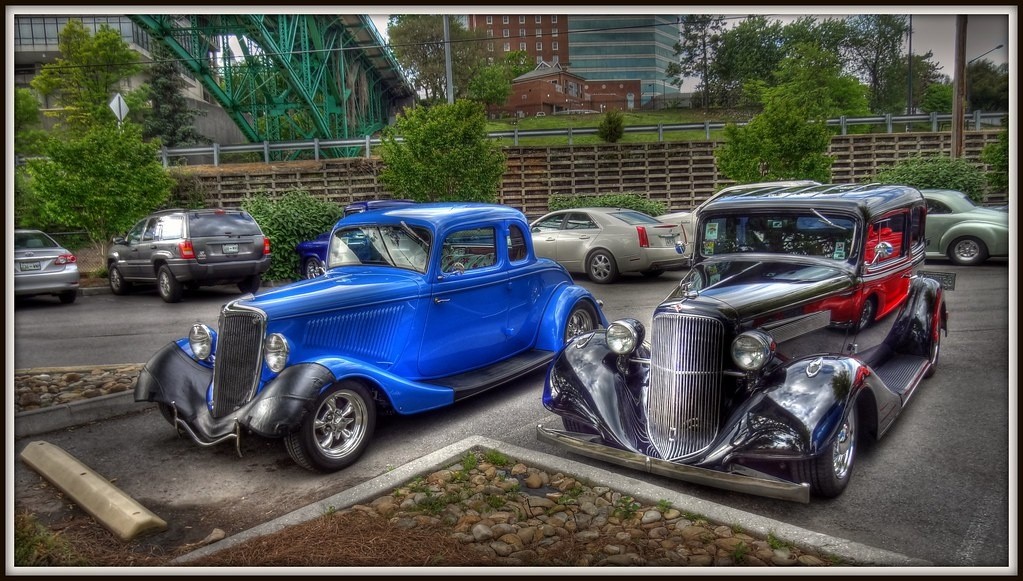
[531,179,952,504]
[14,227,80,304]
[527,207,698,283]
[132,199,614,473]
[816,230,913,328]
[915,188,1008,268]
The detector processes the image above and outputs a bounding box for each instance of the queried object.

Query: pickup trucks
[300,198,521,279]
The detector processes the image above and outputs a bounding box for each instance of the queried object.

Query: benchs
[407,242,494,273]
[768,228,855,255]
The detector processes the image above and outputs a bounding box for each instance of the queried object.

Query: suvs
[106,206,273,303]
